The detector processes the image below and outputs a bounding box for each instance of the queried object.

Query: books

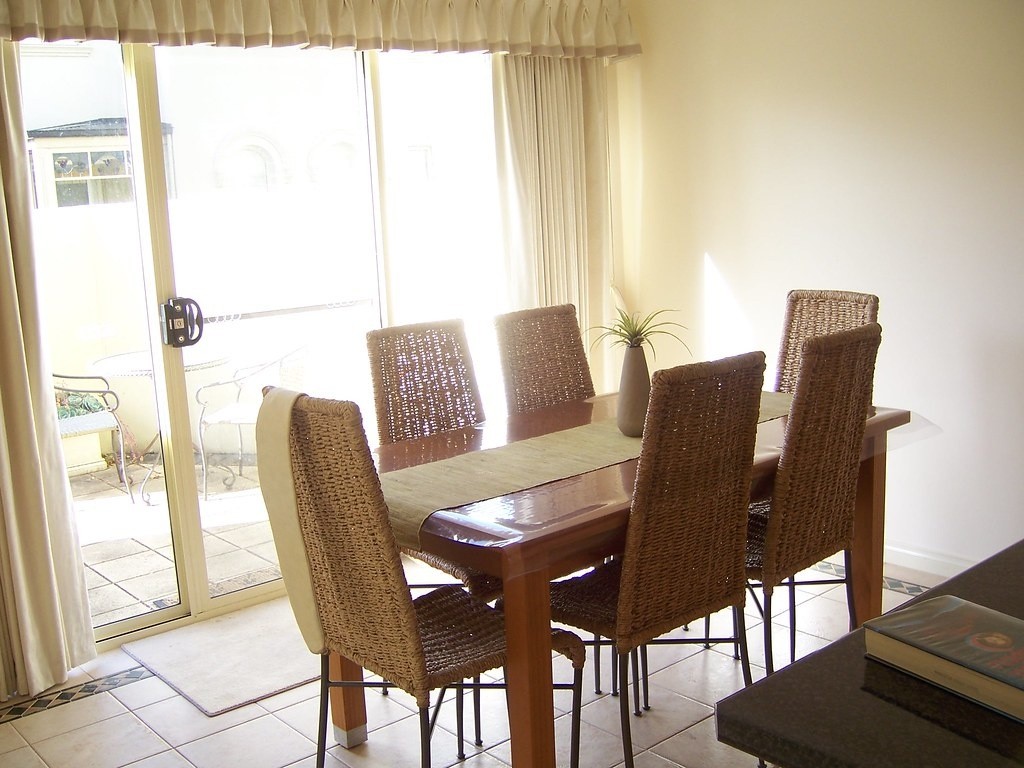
[862,594,1024,723]
[859,654,1024,766]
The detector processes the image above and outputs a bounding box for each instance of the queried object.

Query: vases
[615,347,651,438]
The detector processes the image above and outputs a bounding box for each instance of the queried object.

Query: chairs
[197,357,283,501]
[263,289,883,768]
[51,373,134,504]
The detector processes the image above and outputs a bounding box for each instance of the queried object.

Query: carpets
[120,597,321,717]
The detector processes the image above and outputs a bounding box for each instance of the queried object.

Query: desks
[94,349,235,503]
[717,539,1024,768]
[328,390,910,768]
